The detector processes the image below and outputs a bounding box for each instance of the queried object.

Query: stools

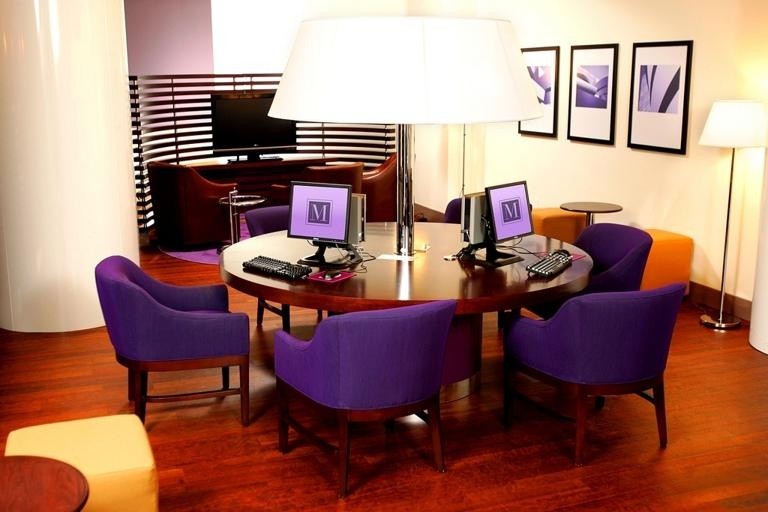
[4,414,159,512]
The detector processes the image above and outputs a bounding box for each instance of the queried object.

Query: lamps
[267,14,544,257]
[697,97,768,329]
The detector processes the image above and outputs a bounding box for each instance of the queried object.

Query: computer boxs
[461,192,485,244]
[348,193,367,244]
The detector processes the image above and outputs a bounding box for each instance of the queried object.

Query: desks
[0,455,90,511]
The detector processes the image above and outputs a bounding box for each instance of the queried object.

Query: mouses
[323,271,341,280]
[549,249,570,257]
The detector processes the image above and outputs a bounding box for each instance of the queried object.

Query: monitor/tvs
[210,88,297,162]
[287,180,352,266]
[485,180,534,267]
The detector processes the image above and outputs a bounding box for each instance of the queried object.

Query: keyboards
[243,255,312,279]
[526,252,572,277]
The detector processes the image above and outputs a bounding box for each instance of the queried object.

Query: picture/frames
[518,46,560,137]
[566,43,618,144]
[628,41,693,156]
